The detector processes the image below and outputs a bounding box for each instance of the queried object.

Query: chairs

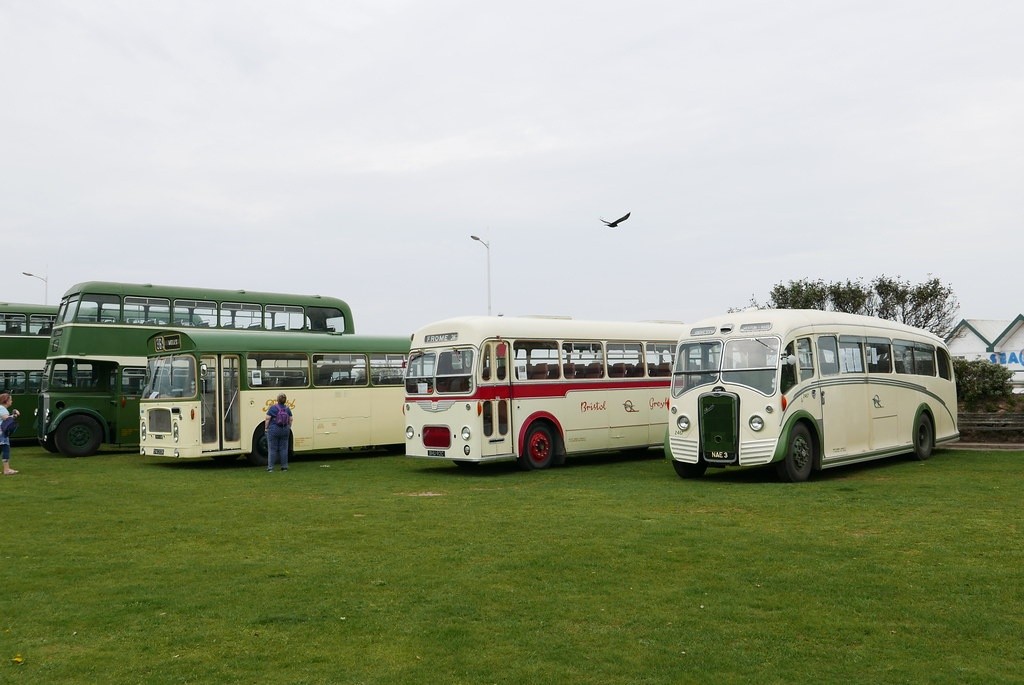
[0,317,949,401]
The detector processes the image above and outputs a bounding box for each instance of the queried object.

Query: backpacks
[274,403,290,426]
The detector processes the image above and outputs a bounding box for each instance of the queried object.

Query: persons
[0,393,18,475]
[264,393,293,473]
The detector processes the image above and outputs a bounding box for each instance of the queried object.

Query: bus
[401,314,747,471]
[0,303,211,439]
[664,308,960,483]
[138,328,415,467]
[34,280,353,458]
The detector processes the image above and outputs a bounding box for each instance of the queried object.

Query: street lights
[470,234,493,315]
[22,272,47,306]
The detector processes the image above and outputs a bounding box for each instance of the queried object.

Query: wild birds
[600,211,631,227]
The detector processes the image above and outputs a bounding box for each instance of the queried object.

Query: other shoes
[4,469,19,475]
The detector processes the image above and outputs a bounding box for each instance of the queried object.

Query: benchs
[956,412,1024,431]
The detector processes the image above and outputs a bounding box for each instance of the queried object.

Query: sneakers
[281,468,287,472]
[267,469,273,473]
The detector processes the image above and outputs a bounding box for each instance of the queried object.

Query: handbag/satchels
[1,416,20,438]
